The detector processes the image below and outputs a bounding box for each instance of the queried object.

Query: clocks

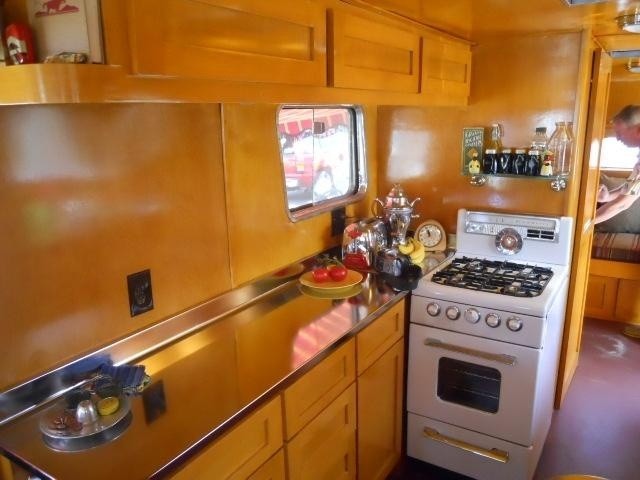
[415,219,447,254]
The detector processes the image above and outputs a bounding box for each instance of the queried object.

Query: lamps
[614,14,640,35]
[625,63,639,74]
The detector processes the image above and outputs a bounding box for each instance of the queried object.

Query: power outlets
[126,268,153,317]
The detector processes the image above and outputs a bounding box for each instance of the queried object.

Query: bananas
[397,236,424,264]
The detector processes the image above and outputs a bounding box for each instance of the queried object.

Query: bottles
[483,122,570,177]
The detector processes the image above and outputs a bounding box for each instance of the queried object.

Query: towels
[94,363,149,396]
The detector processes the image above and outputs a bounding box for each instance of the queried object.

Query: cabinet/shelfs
[0,0,472,110]
[280,335,359,480]
[153,394,290,479]
[352,295,409,480]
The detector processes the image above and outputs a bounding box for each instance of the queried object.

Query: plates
[299,268,364,290]
[38,389,131,440]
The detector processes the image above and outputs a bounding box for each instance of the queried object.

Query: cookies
[96,396,120,415]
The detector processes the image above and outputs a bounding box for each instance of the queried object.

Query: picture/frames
[27,1,105,63]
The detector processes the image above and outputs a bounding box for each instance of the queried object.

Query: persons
[591,104,640,229]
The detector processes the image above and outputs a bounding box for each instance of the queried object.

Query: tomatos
[328,265,348,281]
[312,267,330,281]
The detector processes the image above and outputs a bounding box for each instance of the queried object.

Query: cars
[279,126,350,196]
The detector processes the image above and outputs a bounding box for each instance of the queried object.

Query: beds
[583,171,640,326]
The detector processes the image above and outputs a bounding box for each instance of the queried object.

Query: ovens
[406,295,545,447]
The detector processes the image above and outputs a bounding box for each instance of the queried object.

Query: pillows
[596,196,640,233]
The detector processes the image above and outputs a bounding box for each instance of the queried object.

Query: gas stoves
[409,252,571,318]
